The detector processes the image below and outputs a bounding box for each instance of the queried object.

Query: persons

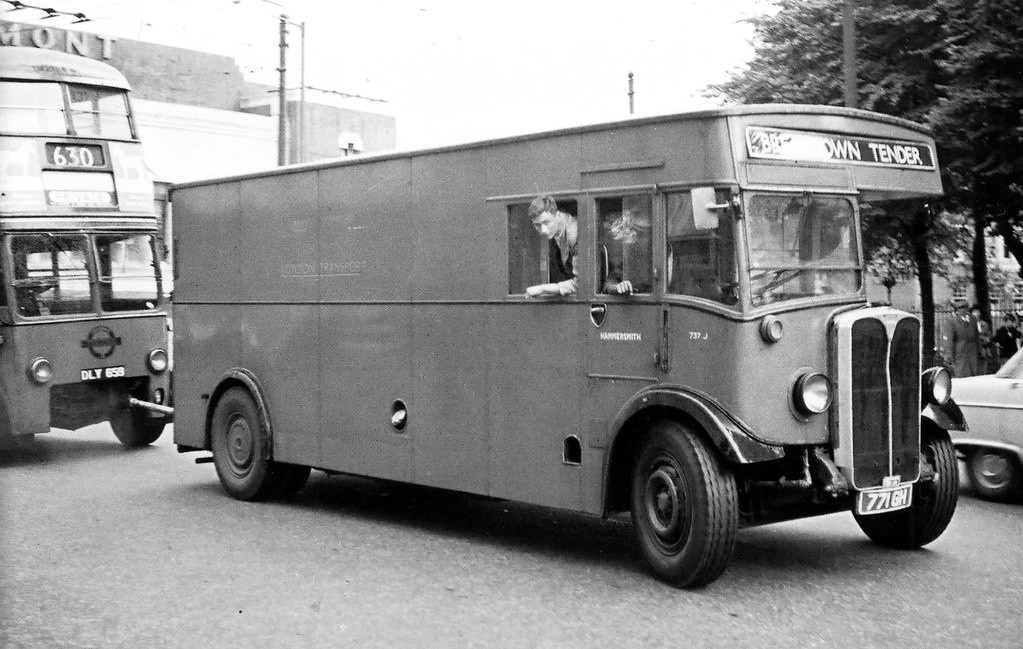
[601,266,633,296]
[945,301,1023,379]
[523,197,579,300]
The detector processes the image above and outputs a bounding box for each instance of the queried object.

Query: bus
[0,42,170,452]
[158,101,970,590]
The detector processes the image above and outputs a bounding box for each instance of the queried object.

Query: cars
[948,346,1023,502]
